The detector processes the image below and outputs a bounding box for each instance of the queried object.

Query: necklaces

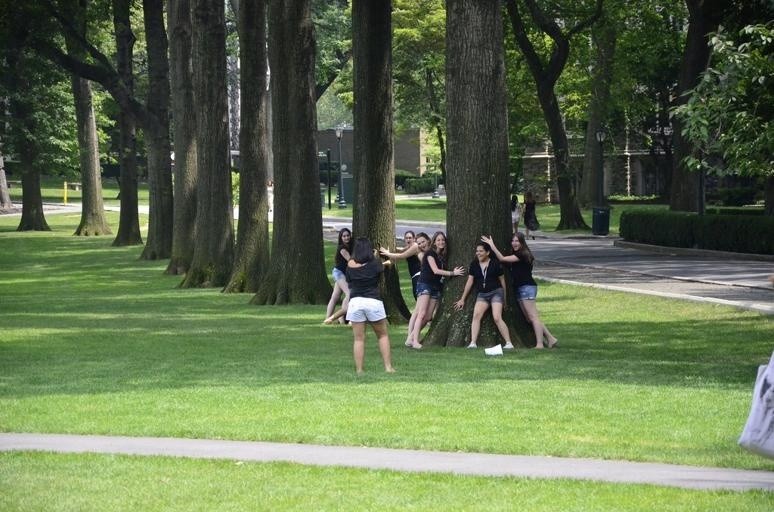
[480,258,490,289]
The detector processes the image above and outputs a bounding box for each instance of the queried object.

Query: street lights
[595,122,607,205]
[335,127,348,209]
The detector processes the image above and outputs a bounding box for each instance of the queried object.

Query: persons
[451,243,515,350]
[479,230,558,350]
[522,191,540,240]
[511,194,522,233]
[322,227,465,377]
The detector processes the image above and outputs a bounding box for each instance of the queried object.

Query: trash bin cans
[592,207,611,235]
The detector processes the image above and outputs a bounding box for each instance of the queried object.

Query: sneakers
[503,342,514,349]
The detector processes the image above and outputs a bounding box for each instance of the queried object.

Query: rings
[458,306,460,307]
